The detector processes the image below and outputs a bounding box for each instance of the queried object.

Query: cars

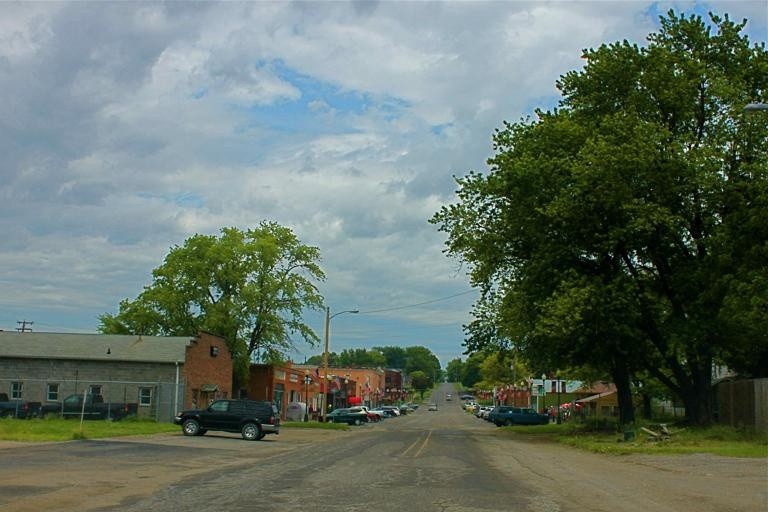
[459,395,550,426]
[325,402,419,426]
[428,394,452,411]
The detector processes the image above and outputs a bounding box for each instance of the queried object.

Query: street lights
[322,306,359,423]
[303,369,312,423]
[500,367,561,425]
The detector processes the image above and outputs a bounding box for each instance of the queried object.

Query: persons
[327,402,332,414]
[307,403,313,422]
[550,406,556,423]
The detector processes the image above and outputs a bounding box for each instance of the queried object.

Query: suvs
[173,399,281,441]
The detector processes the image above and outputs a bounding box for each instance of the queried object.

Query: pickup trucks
[39,393,138,422]
[0,392,42,420]
